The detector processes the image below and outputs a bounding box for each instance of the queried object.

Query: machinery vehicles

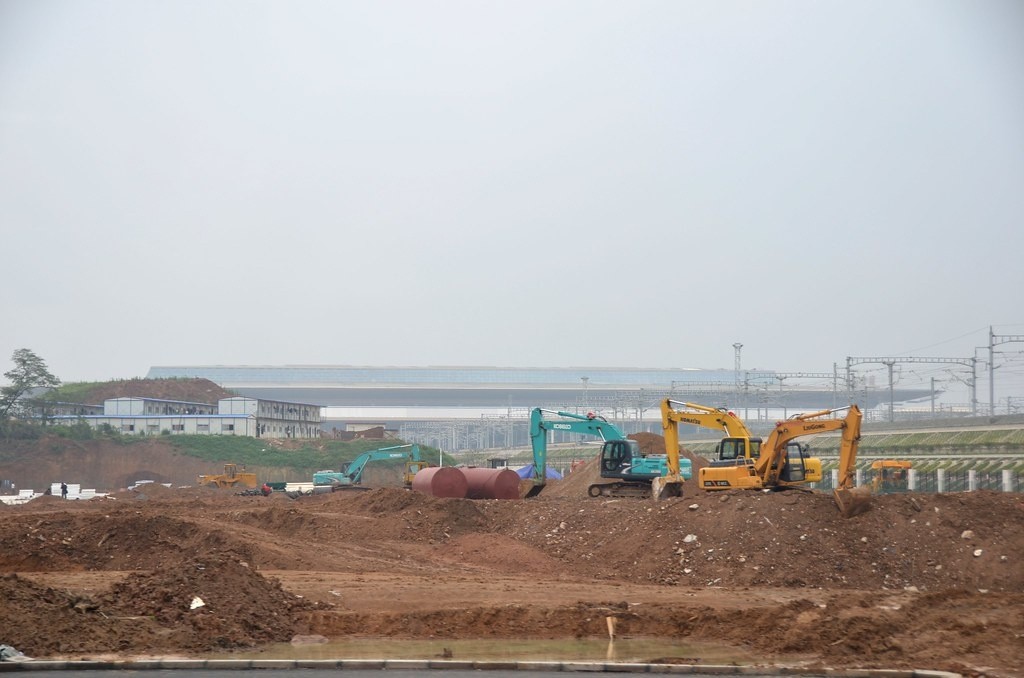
[651,396,823,502]
[860,460,912,492]
[402,462,429,489]
[698,404,872,517]
[196,464,257,493]
[518,408,693,500]
[312,444,422,493]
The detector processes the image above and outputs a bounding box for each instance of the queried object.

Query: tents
[519,463,561,480]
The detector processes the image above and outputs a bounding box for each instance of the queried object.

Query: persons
[61,482,67,498]
[11,481,16,494]
